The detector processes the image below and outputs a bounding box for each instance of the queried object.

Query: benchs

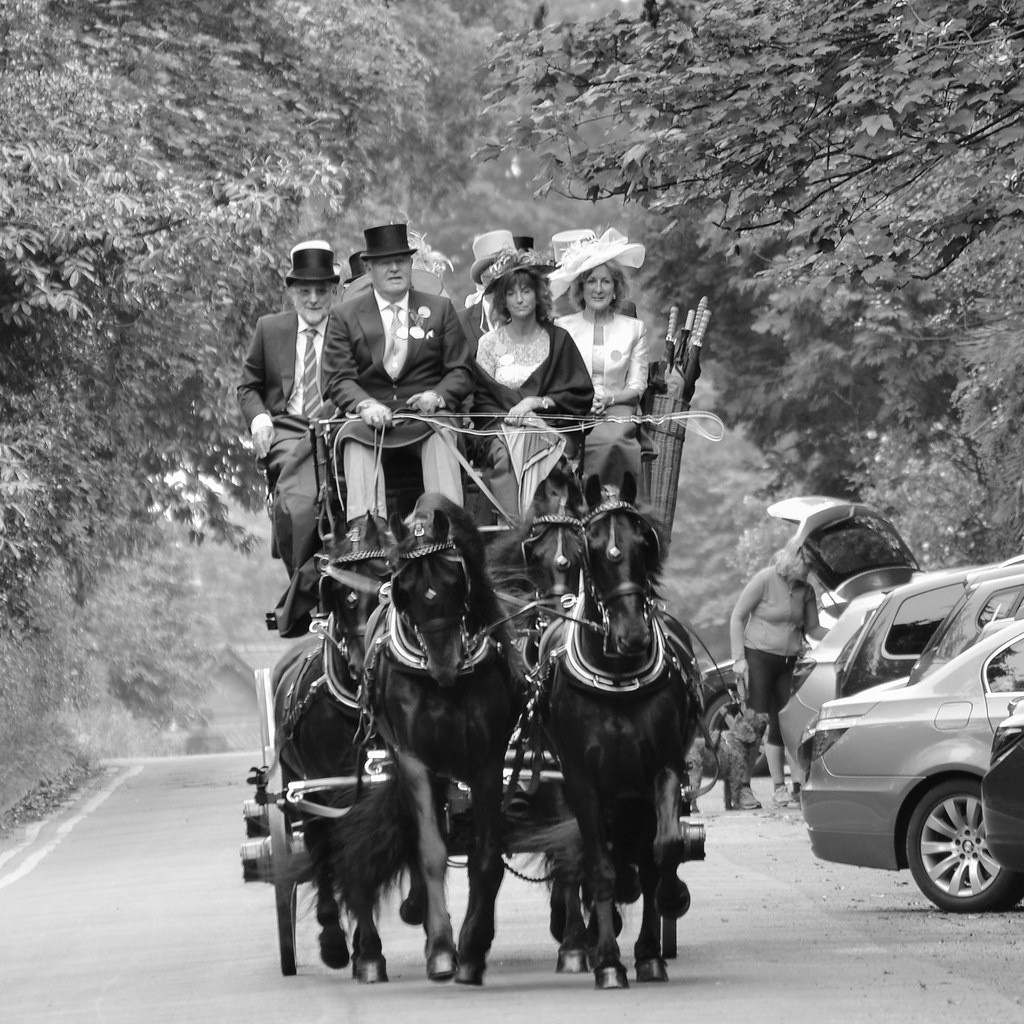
[326,408,589,532]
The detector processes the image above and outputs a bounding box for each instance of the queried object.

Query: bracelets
[609,393,615,406]
[432,391,443,407]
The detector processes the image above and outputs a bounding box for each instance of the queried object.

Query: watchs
[540,395,550,410]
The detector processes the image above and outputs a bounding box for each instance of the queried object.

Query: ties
[302,329,321,419]
[387,305,403,340]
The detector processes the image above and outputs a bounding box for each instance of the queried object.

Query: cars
[799,617,1024,914]
[702,657,772,783]
[979,712,1024,873]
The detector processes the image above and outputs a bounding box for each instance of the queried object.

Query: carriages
[239,473,707,992]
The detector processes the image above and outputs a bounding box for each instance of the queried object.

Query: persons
[236,224,652,544]
[729,536,829,811]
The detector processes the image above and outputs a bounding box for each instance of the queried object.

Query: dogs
[683,708,770,813]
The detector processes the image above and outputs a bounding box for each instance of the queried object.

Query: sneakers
[734,787,761,809]
[772,785,794,807]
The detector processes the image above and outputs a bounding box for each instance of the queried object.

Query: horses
[520,470,699,992]
[274,493,427,986]
[366,491,533,987]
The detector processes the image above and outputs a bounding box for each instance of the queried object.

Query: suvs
[835,563,975,707]
[906,554,1024,687]
[778,584,902,781]
[768,496,921,653]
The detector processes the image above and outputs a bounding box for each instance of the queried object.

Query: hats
[359,223,417,261]
[465,230,562,309]
[284,239,342,289]
[547,226,646,302]
[342,251,444,295]
